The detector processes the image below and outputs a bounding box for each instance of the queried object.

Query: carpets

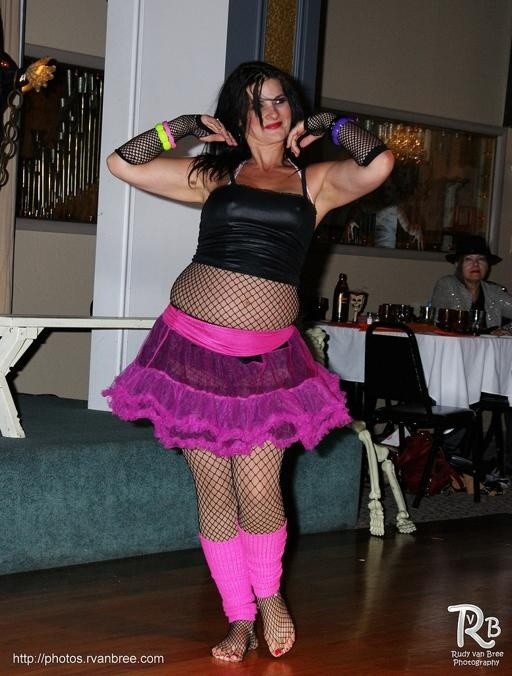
[357,483,512,530]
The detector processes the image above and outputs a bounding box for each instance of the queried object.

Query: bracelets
[161,120,177,150]
[155,122,171,152]
[327,114,348,140]
[331,115,358,146]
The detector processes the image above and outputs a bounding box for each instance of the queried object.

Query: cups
[420,305,435,323]
[377,303,414,323]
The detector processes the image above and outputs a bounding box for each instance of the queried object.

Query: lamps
[377,122,431,168]
[0,54,59,190]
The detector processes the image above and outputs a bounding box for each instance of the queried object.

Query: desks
[0,314,160,440]
[307,321,512,504]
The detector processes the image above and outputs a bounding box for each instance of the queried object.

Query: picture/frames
[311,93,507,264]
[12,43,105,236]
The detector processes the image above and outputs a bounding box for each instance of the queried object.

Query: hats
[445,236,502,265]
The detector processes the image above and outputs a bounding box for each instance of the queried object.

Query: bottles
[366,312,373,324]
[331,271,349,324]
[437,308,486,336]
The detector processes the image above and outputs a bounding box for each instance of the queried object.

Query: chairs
[364,321,482,508]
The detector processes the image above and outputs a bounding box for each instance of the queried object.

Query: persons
[103,61,396,664]
[427,235,511,339]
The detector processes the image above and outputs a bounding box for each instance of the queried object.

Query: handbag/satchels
[395,430,465,498]
